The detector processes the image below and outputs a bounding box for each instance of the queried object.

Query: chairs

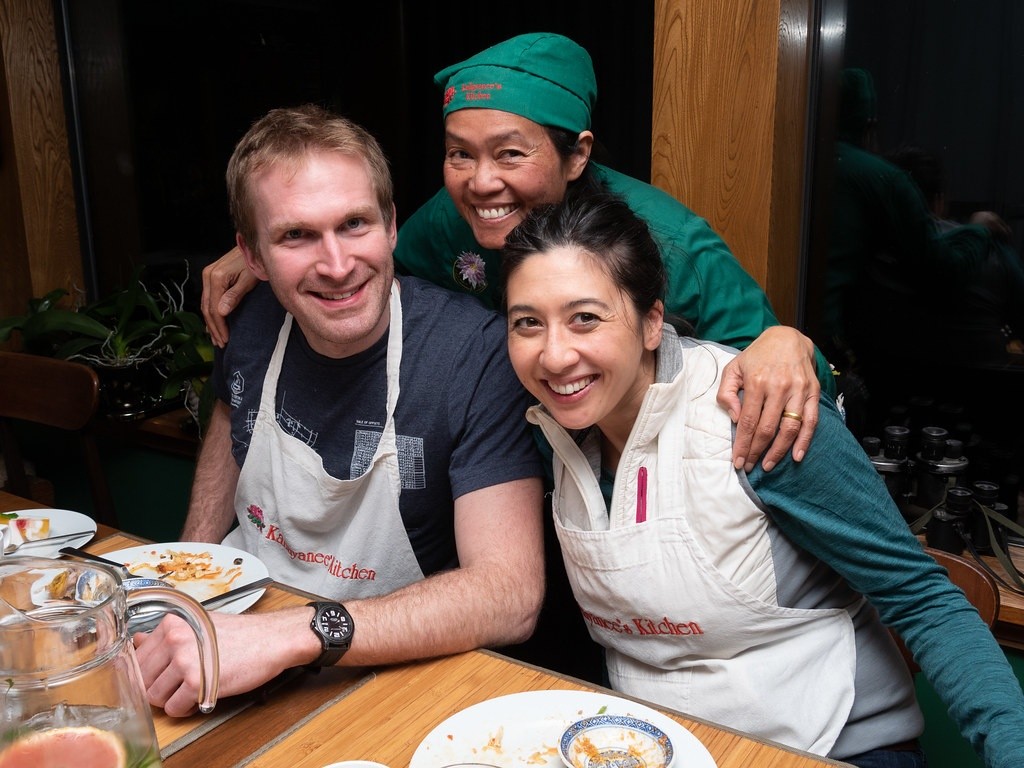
[0,352,121,531]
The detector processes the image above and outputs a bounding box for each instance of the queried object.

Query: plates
[119,577,172,624]
[0,502,98,566]
[557,715,674,768]
[75,570,116,606]
[32,541,269,631]
[408,689,718,768]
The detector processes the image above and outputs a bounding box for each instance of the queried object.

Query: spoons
[5,529,95,556]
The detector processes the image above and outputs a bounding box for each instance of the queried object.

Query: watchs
[304,601,356,675]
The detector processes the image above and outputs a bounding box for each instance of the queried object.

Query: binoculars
[926,480,1009,557]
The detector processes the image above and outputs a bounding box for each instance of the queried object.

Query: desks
[0,488,858,768]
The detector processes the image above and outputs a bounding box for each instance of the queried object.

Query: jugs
[0,559,222,768]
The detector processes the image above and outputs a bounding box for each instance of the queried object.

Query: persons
[198,33,838,476]
[128,104,548,723]
[496,191,1024,768]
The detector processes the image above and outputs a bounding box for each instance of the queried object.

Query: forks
[62,545,174,581]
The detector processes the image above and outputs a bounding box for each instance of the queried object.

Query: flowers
[456,250,488,290]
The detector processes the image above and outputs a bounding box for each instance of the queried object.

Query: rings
[781,412,804,423]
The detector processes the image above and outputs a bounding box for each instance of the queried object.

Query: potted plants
[0,260,217,433]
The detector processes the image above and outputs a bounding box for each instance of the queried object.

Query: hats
[433,33,598,132]
